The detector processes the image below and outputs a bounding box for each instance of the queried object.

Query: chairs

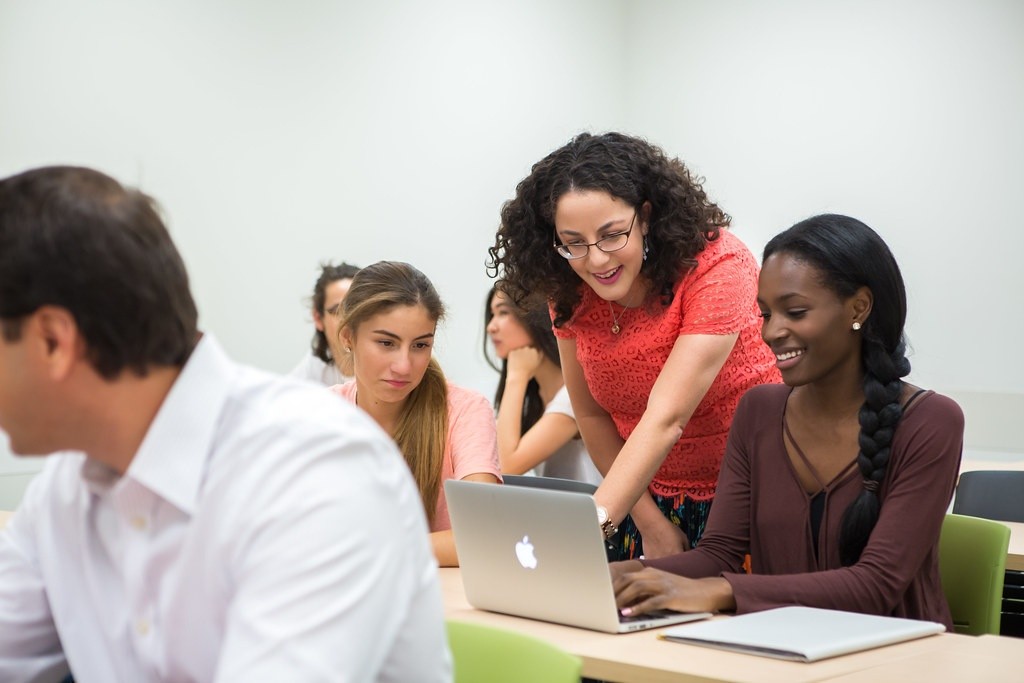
[938,509,1012,639]
[953,470,1024,524]
[444,620,582,683]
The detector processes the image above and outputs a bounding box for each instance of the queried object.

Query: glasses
[321,304,342,315]
[552,206,640,260]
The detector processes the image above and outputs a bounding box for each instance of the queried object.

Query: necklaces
[609,281,642,334]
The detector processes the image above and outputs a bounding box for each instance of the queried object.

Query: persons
[326,261,504,568]
[289,263,362,390]
[483,273,605,489]
[607,213,963,634]
[484,132,787,560]
[0,166,453,683]
[490,131,783,561]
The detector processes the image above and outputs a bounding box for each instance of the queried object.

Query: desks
[997,521,1024,571]
[440,564,980,683]
[820,632,1024,683]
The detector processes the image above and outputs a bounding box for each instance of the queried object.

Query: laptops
[443,479,713,634]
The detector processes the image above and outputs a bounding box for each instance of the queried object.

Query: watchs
[596,505,616,539]
